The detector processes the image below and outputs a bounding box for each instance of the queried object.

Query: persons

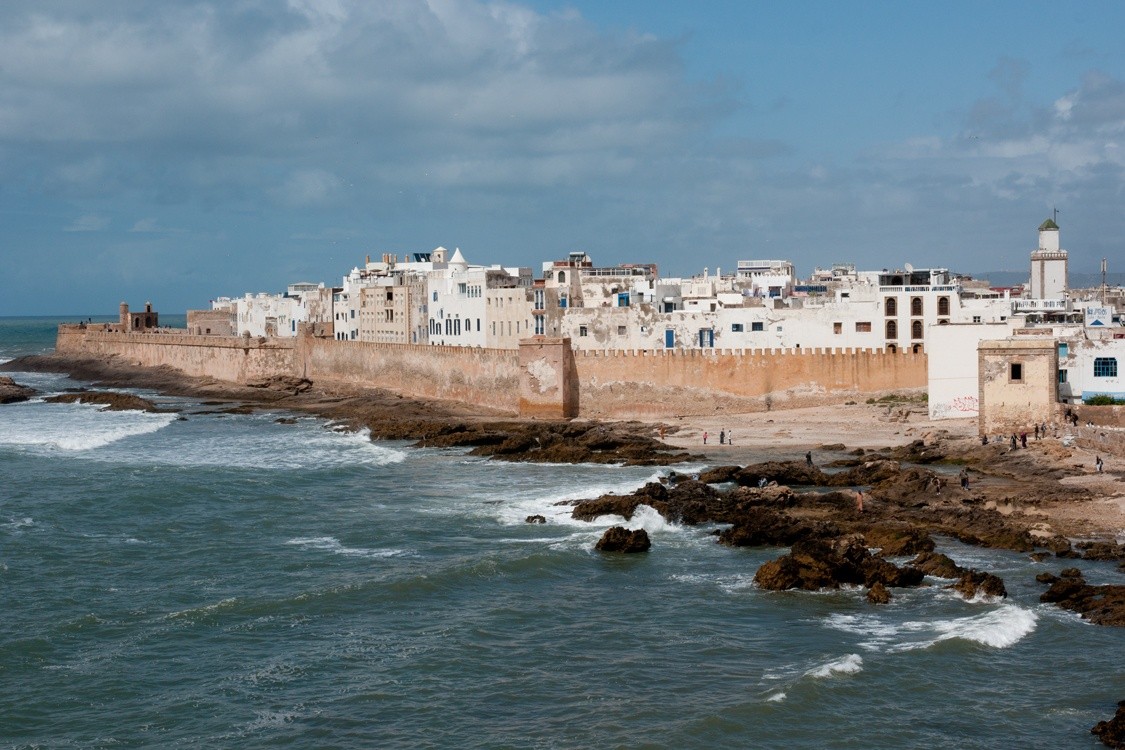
[983,422,1046,450]
[1095,456,1104,471]
[1071,412,1094,427]
[660,423,665,439]
[856,490,863,513]
[600,425,612,433]
[703,428,732,445]
[933,468,969,495]
[805,451,812,467]
[758,476,768,488]
[80,317,91,325]
